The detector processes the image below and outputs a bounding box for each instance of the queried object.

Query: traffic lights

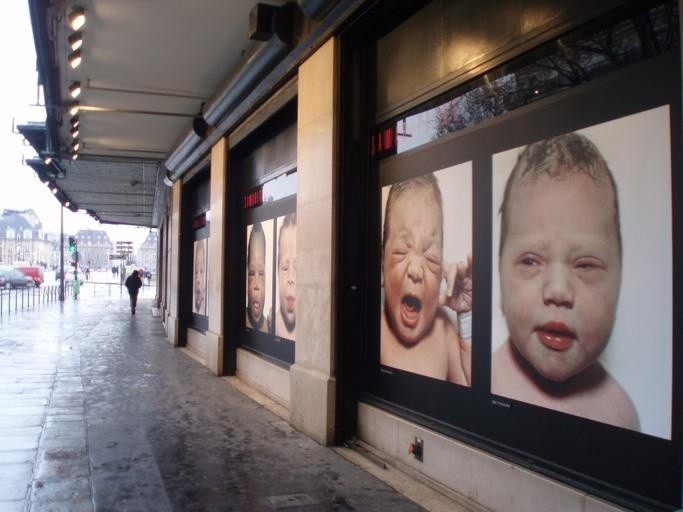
[69,236,76,252]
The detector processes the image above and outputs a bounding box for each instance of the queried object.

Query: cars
[0,264,45,289]
[55,264,86,286]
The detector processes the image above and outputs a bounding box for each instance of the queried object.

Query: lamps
[68,116,80,161]
[67,5,85,99]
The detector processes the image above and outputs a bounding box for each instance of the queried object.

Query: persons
[194,238,205,311]
[490,134,639,434]
[379,173,472,388]
[277,214,298,342]
[124,271,141,315]
[248,219,265,335]
